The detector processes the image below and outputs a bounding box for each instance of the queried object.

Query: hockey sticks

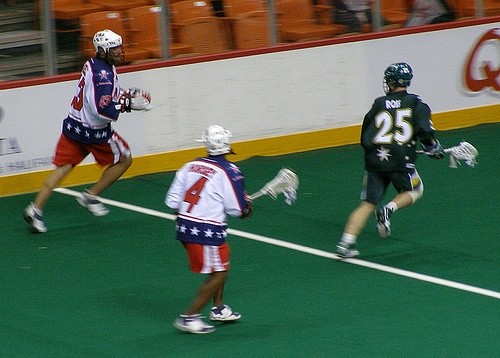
[414,141,480,164]
[246,167,299,201]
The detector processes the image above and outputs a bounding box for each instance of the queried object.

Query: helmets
[93,28,125,67]
[383,62,413,96]
[194,124,232,156]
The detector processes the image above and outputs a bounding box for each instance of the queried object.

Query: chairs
[36,0,500,64]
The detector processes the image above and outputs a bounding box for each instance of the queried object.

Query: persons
[164,125,252,334]
[334,63,444,259]
[24,28,153,232]
[405,0,455,27]
[333,0,385,33]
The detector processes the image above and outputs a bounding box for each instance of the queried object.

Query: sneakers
[174,312,216,333]
[375,205,394,238]
[75,188,110,216]
[336,240,360,257]
[24,201,47,233]
[210,305,241,321]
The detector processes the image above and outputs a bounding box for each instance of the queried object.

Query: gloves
[120,88,151,113]
[424,139,446,160]
